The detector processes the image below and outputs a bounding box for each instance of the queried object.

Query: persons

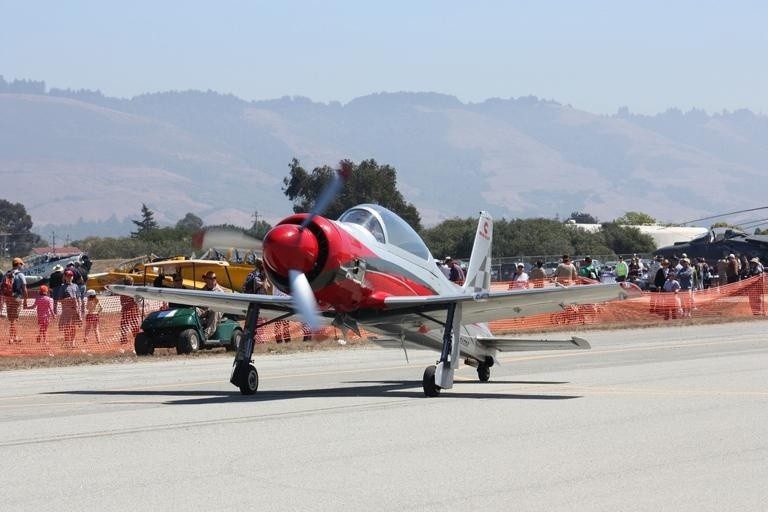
[511,253,764,322]
[443,255,465,282]
[153,255,313,344]
[0,255,147,347]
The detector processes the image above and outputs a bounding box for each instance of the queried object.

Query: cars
[433,254,670,292]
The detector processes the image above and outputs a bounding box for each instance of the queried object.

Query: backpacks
[242,271,257,293]
[0,270,22,298]
[583,267,600,283]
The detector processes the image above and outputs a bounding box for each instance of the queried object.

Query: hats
[749,258,759,265]
[517,262,524,269]
[619,253,705,275]
[40,261,97,297]
[12,258,25,265]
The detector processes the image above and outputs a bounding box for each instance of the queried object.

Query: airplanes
[98,158,642,402]
[0,247,269,300]
[648,224,767,272]
